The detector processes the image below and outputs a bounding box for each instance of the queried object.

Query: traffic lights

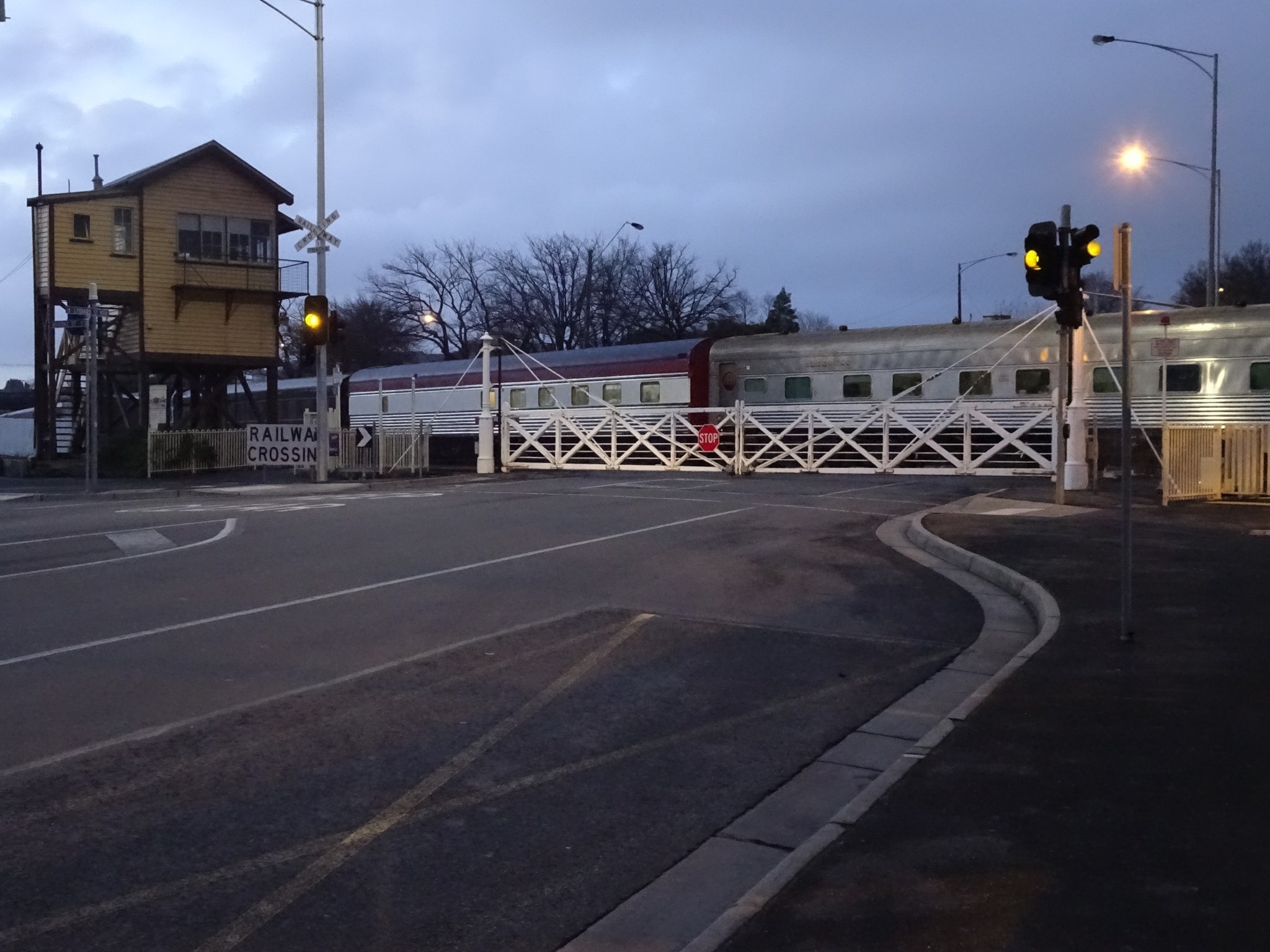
[1072,223,1100,270]
[1021,220,1056,297]
[1053,290,1083,329]
[303,295,333,347]
[330,309,349,343]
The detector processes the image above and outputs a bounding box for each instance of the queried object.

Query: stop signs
[697,425,720,452]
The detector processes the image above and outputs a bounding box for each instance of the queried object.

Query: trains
[177,302,1270,470]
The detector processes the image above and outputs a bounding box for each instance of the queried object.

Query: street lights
[1092,35,1216,309]
[1120,146,1222,306]
[958,252,1019,323]
